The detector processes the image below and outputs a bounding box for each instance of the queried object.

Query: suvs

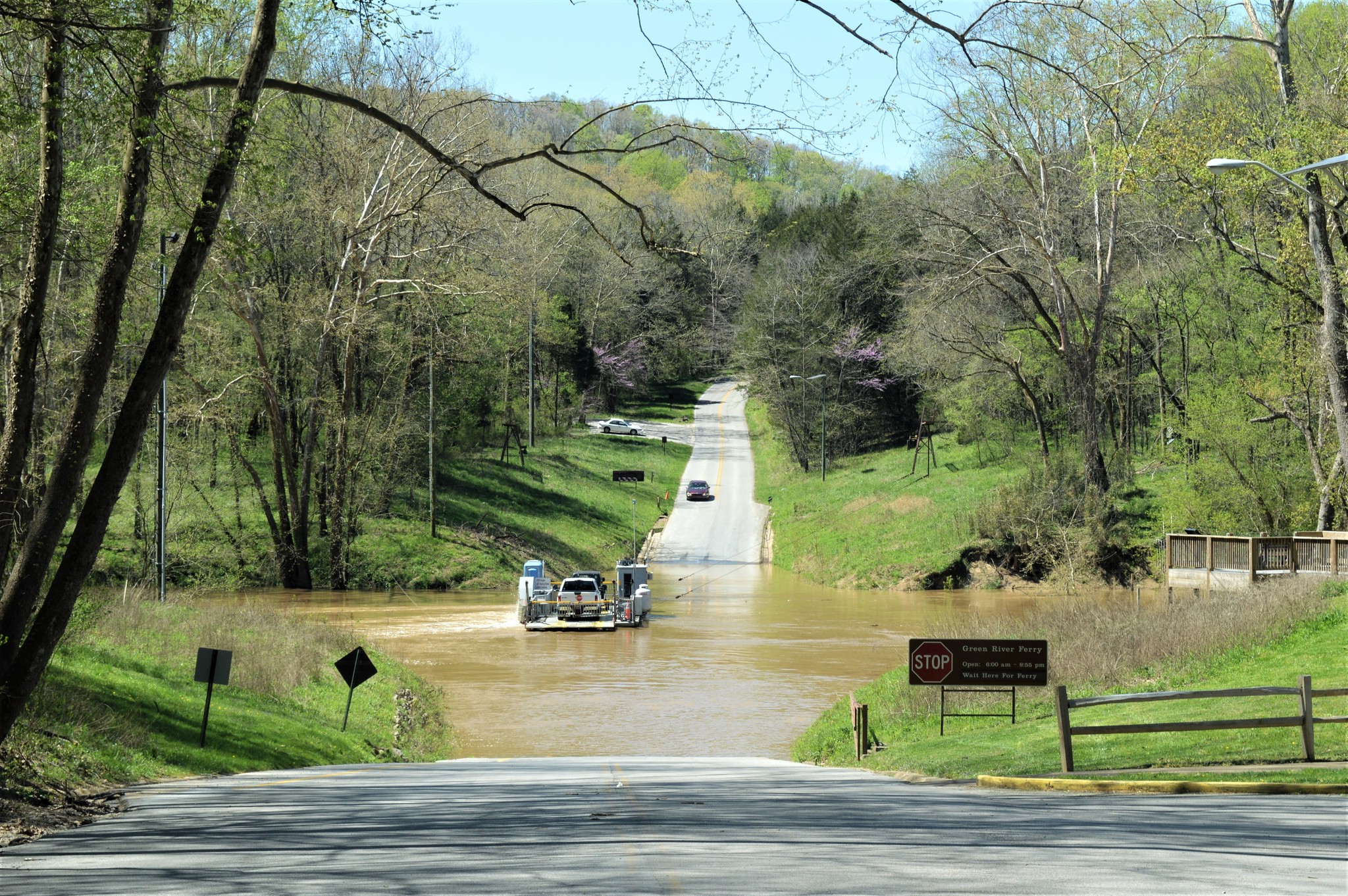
[552,571,607,620]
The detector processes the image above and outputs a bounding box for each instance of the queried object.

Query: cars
[598,418,644,435]
[685,480,710,500]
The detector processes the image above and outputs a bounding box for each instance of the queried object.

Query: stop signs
[912,642,953,683]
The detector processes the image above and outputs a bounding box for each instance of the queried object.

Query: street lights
[790,374,827,483]
